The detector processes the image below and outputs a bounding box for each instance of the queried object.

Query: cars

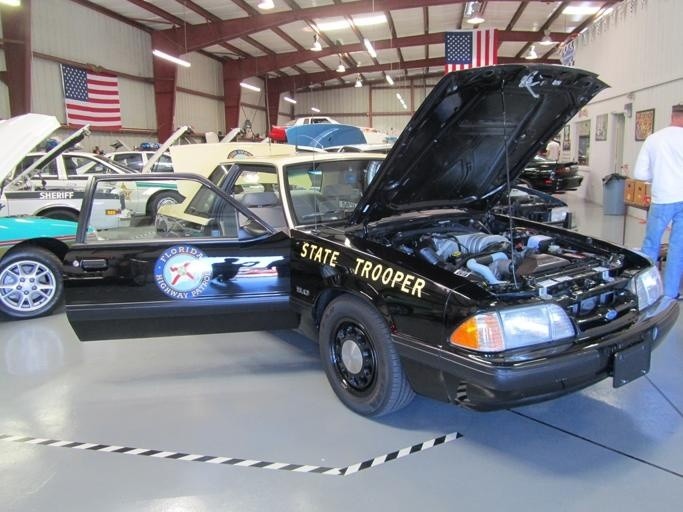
[500,177,571,229]
[1,111,584,323]
[59,62,681,421]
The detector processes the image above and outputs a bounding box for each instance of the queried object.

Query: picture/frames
[595,113,608,142]
[634,108,655,141]
[564,125,571,151]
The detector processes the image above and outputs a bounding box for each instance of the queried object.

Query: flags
[443,29,497,76]
[58,63,122,130]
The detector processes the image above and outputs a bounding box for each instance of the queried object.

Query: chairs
[319,184,359,222]
[238,193,288,231]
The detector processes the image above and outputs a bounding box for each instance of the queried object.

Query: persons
[540,139,559,161]
[632,105,683,299]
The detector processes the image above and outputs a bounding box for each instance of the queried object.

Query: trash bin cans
[602,173,629,215]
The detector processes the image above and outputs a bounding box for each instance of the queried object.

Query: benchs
[220,190,322,236]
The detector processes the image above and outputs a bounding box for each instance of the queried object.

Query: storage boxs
[623,179,651,210]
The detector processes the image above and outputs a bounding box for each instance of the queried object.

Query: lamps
[539,29,553,45]
[466,0,485,24]
[525,45,539,59]
[152,0,407,112]
